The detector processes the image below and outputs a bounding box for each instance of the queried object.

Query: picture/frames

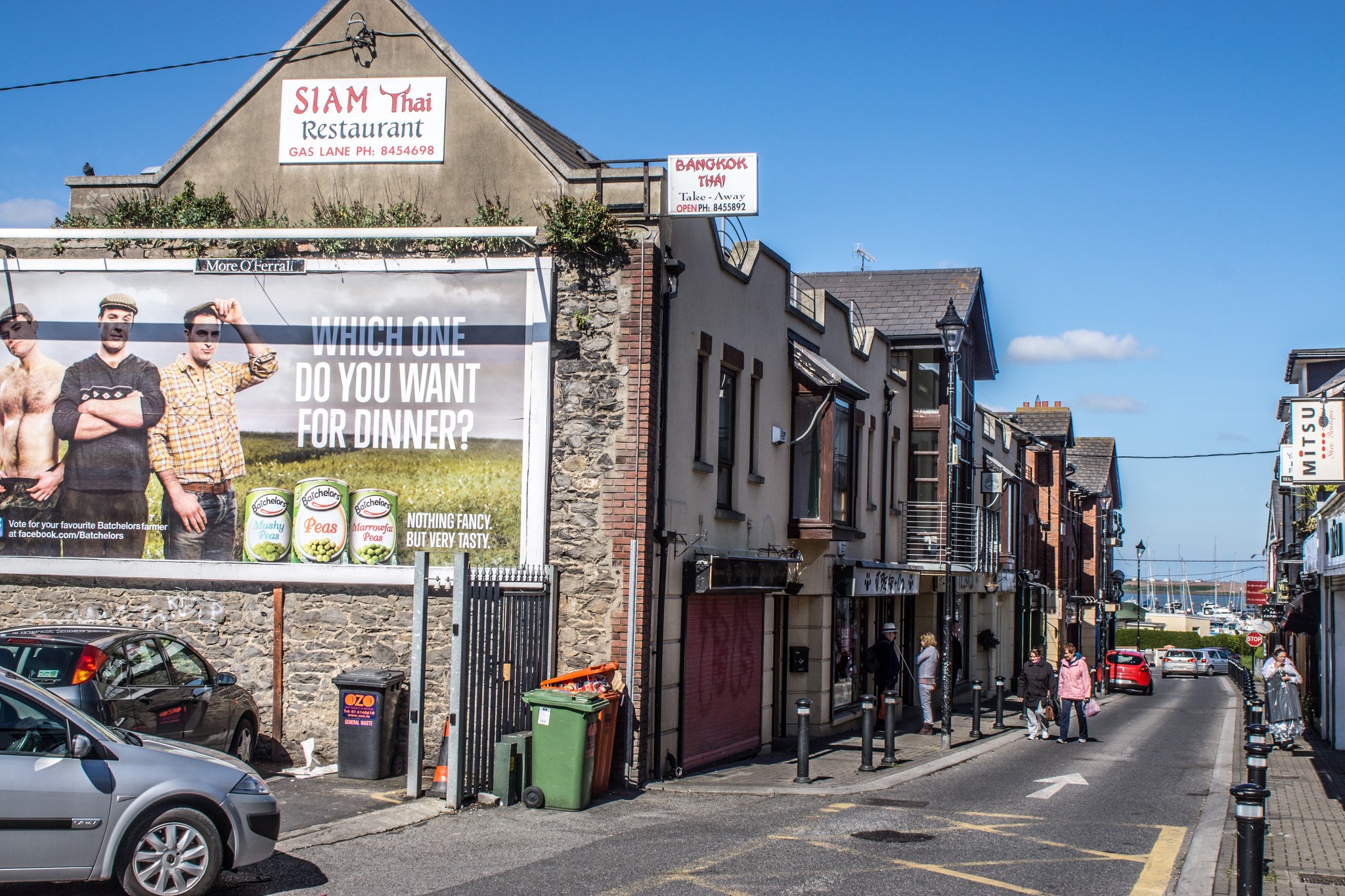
[980,471,1003,494]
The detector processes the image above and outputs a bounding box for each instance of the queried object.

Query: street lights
[934,296,969,749]
[1134,540,1146,653]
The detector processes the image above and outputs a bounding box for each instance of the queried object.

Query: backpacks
[861,639,892,674]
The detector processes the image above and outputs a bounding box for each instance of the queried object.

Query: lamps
[1071,596,1097,604]
[1002,569,1053,596]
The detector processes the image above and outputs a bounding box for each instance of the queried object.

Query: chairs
[0,648,147,686]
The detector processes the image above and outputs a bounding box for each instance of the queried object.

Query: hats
[184,300,222,321]
[880,623,900,634]
[1,303,33,324]
[99,291,139,317]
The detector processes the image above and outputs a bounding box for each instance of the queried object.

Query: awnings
[1277,591,1321,633]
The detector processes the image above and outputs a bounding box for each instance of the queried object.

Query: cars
[1203,647,1240,663]
[1192,649,1234,676]
[1096,649,1154,697]
[1159,648,1198,679]
[0,663,282,895]
[0,625,262,767]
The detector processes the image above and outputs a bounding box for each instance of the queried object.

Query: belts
[179,479,232,496]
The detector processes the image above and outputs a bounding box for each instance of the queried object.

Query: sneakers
[1056,738,1068,744]
[1042,731,1049,739]
[1027,734,1039,740]
[1283,743,1295,750]
[1078,738,1087,742]
[1272,741,1280,750]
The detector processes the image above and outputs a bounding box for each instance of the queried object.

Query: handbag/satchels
[1045,695,1055,722]
[1048,690,1063,727]
[877,687,888,720]
[1082,698,1101,718]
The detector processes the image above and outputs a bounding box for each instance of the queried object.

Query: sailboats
[1124,537,1272,636]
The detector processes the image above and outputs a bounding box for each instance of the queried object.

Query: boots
[914,722,934,735]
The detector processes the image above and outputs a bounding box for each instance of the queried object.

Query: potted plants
[983,567,999,593]
[783,545,807,596]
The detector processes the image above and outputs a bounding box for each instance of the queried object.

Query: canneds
[243,477,398,565]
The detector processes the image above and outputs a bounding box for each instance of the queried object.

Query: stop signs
[1246,631,1263,647]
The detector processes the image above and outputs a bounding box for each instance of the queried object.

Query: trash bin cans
[520,687,611,813]
[331,668,406,780]
[539,661,628,800]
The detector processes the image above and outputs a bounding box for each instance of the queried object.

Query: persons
[951,619,963,714]
[54,294,167,559]
[913,632,940,733]
[1,303,75,559]
[1056,642,1092,744]
[151,299,282,565]
[1261,645,1295,672]
[1016,646,1057,740]
[1265,649,1302,750]
[874,622,901,737]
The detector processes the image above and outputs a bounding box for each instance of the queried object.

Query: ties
[954,635,959,644]
